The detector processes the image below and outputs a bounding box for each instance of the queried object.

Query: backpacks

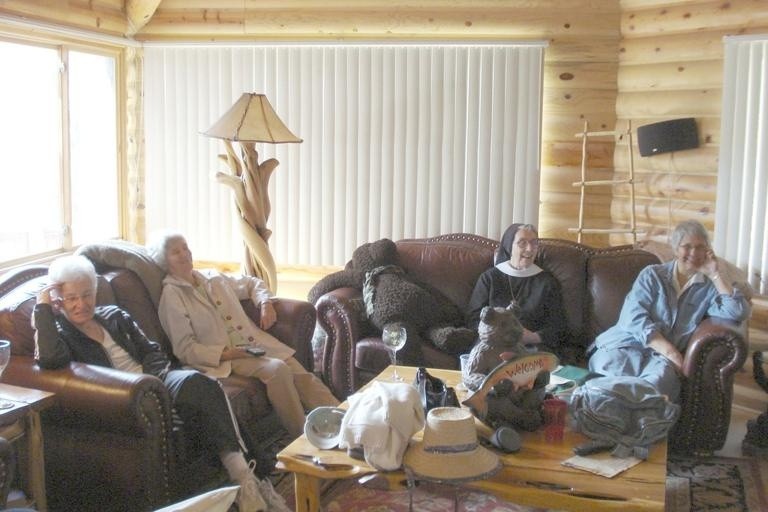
[571,374,684,458]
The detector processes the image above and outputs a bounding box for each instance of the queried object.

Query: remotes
[572,439,616,456]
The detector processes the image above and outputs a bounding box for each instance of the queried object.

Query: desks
[0,382,56,512]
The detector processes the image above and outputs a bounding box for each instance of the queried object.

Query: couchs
[315,233,749,458]
[0,249,317,512]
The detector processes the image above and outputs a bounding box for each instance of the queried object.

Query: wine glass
[0,340,14,409]
[379,324,407,382]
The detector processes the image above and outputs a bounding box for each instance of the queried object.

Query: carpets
[274,454,768,512]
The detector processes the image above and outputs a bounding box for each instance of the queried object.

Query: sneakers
[259,479,292,512]
[234,479,267,511]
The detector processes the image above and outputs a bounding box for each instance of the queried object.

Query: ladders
[570,119,645,249]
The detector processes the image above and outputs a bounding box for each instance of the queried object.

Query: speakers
[638,118,698,156]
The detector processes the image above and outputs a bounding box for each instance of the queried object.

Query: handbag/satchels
[412,363,461,415]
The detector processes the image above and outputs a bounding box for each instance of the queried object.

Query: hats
[303,405,344,450]
[403,405,505,482]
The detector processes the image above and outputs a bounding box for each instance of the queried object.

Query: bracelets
[260,299,270,303]
[710,273,723,284]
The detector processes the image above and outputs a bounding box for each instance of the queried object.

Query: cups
[543,398,565,441]
[460,353,470,379]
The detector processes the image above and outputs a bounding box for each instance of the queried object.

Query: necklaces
[506,269,531,303]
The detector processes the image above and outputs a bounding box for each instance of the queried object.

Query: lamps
[199,93,305,299]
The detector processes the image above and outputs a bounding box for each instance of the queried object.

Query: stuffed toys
[463,304,560,430]
[307,238,473,367]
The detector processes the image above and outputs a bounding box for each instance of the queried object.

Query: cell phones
[246,347,265,355]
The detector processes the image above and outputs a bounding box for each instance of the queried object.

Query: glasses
[513,238,540,250]
[54,293,93,308]
[679,243,709,253]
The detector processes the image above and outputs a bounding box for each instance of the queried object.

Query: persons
[586,220,754,391]
[466,222,569,347]
[145,228,346,444]
[33,254,290,512]
